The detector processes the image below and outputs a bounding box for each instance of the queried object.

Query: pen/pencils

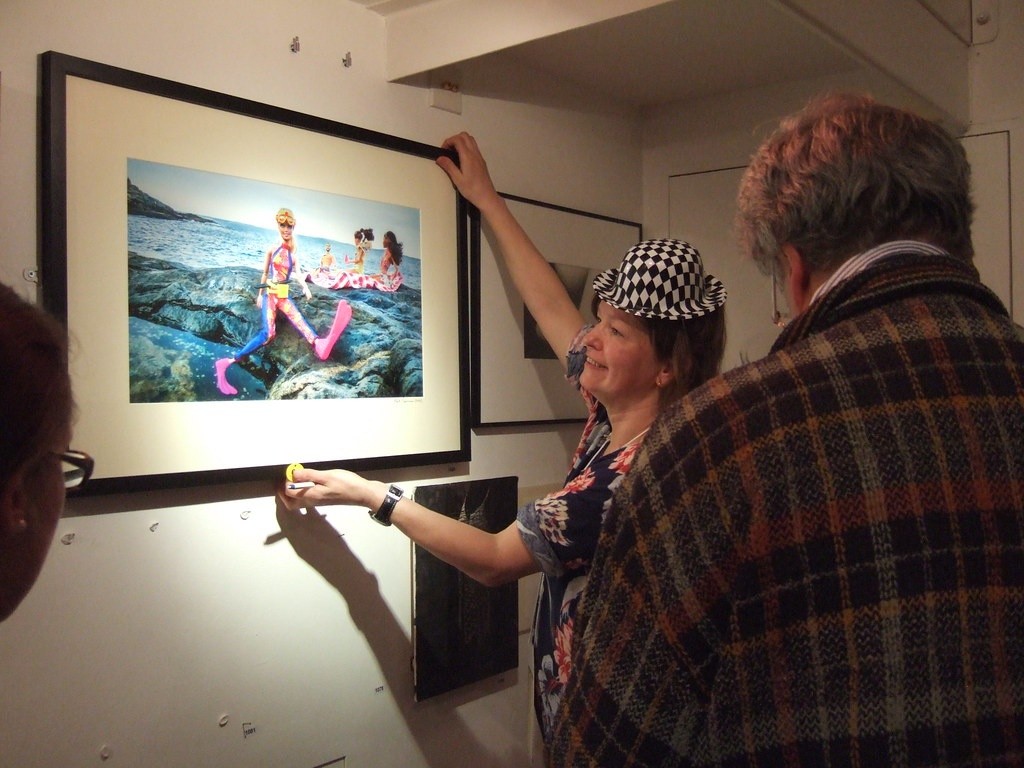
[287,482,315,489]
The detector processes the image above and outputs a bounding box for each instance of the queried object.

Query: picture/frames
[471,190,646,427]
[38,51,472,500]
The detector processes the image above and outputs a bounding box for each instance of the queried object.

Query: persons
[0,282,98,636]
[533,91,1024,768]
[210,205,407,397]
[274,130,729,768]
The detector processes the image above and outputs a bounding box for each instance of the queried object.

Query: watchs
[368,479,405,527]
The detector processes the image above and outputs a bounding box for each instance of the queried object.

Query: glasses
[771,256,797,329]
[12,450,93,494]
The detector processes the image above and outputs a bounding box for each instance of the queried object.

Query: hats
[593,238,727,320]
